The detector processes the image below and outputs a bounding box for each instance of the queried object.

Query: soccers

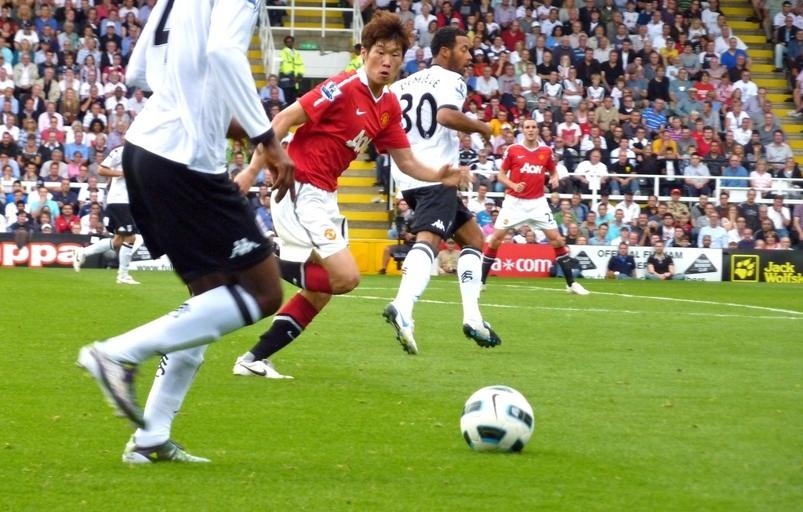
[459,384,535,453]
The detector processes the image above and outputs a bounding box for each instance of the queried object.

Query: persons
[55,204,80,233]
[7,210,33,234]
[72,144,141,285]
[417,61,426,71]
[404,48,428,76]
[414,4,436,32]
[6,180,28,203]
[27,180,53,206]
[406,18,419,40]
[437,2,464,29]
[265,85,283,107]
[231,168,240,180]
[372,156,383,187]
[41,205,49,212]
[418,20,437,46]
[33,211,56,234]
[399,35,420,69]
[79,192,106,218]
[9,200,28,223]
[257,196,274,229]
[260,75,285,101]
[364,141,375,161]
[77,176,104,202]
[377,147,390,194]
[251,185,268,207]
[30,187,60,220]
[52,179,78,207]
[399,0,414,22]
[383,27,502,358]
[595,0,803,248]
[345,44,364,72]
[606,241,636,280]
[459,0,605,245]
[279,35,304,104]
[226,139,248,164]
[80,203,100,226]
[371,0,393,11]
[81,215,99,235]
[379,199,415,274]
[88,222,108,236]
[256,168,273,187]
[40,223,53,233]
[71,222,81,233]
[267,103,281,121]
[449,17,459,29]
[5,190,30,216]
[75,0,284,466]
[437,239,460,275]
[642,240,684,280]
[0,0,154,181]
[482,118,591,293]
[227,152,249,174]
[231,12,477,380]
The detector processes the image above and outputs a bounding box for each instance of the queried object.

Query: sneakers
[462,320,502,348]
[74,341,146,429]
[232,355,294,379]
[122,436,212,463]
[382,302,418,355]
[566,281,590,295]
[117,274,141,285]
[71,246,85,273]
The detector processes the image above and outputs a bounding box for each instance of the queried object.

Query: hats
[670,188,681,194]
[41,223,51,229]
